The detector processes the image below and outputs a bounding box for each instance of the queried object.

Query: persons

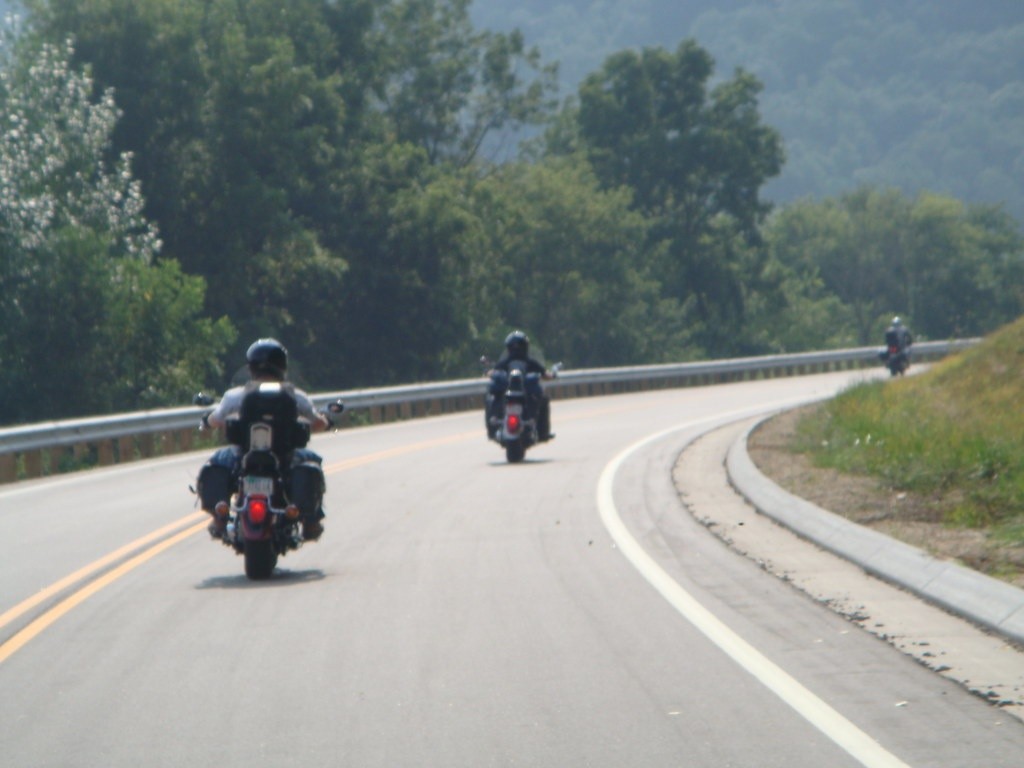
[879,316,913,363]
[201,337,336,540]
[485,330,557,443]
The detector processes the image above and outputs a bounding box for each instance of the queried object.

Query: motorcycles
[479,354,563,464]
[187,390,347,581]
[878,327,912,376]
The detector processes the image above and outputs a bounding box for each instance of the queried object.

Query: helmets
[246,338,289,379]
[505,331,529,356]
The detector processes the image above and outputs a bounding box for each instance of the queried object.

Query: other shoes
[208,521,224,538]
[549,433,555,439]
[302,520,324,540]
